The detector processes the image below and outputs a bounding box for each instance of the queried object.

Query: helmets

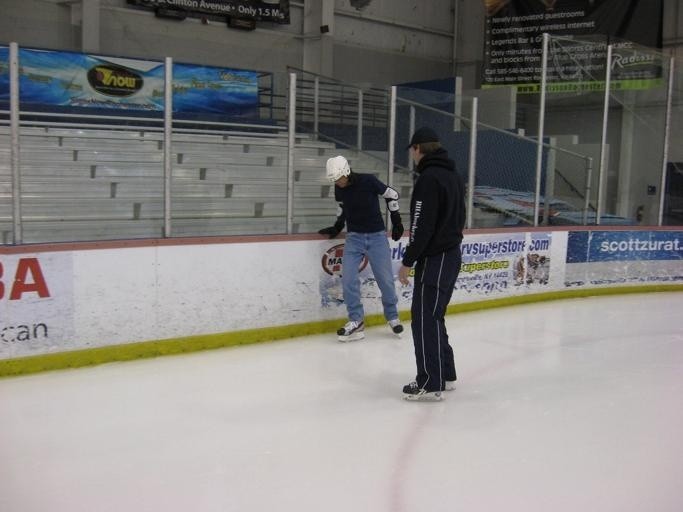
[325,155,351,183]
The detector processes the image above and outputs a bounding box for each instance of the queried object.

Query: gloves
[390,213,404,241]
[318,222,344,239]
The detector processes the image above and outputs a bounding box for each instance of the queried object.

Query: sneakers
[337,320,364,336]
[403,377,445,395]
[387,318,403,334]
[443,373,457,382]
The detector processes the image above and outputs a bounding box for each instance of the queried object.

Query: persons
[399,128,466,394]
[319,155,404,336]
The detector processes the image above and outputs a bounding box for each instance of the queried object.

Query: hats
[405,127,439,149]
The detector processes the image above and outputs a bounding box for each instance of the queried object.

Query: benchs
[0,124,505,245]
